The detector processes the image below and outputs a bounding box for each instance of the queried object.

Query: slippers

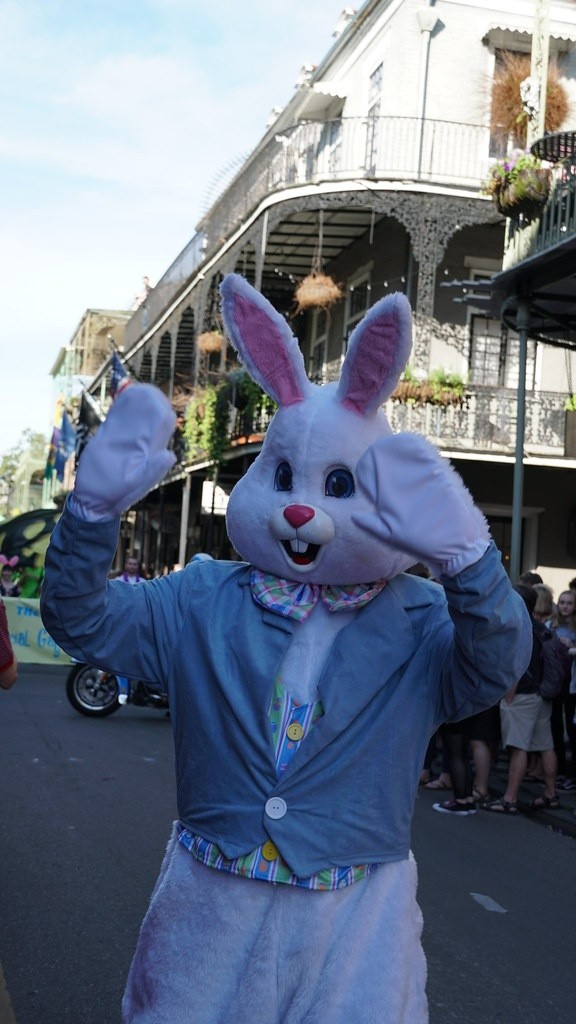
[521,768,544,783]
[424,779,451,791]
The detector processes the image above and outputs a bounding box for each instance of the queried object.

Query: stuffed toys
[39,272,533,1024]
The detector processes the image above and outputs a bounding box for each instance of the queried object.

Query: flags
[44,428,60,480]
[76,391,102,470]
[110,350,131,400]
[54,409,76,482]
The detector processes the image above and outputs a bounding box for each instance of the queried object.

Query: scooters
[65,657,172,718]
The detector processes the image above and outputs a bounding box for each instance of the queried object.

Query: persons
[0,564,21,597]
[109,559,146,697]
[0,595,17,689]
[416,569,576,815]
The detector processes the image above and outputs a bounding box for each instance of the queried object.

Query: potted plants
[393,364,466,408]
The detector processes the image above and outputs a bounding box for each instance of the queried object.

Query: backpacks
[527,629,564,700]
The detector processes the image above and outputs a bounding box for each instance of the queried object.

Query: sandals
[472,787,490,803]
[527,791,560,810]
[480,797,518,815]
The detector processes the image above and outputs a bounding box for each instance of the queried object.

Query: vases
[493,167,552,215]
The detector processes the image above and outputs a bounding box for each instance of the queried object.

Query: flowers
[481,146,569,198]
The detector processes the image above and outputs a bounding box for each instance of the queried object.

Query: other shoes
[432,800,476,816]
[539,777,576,793]
